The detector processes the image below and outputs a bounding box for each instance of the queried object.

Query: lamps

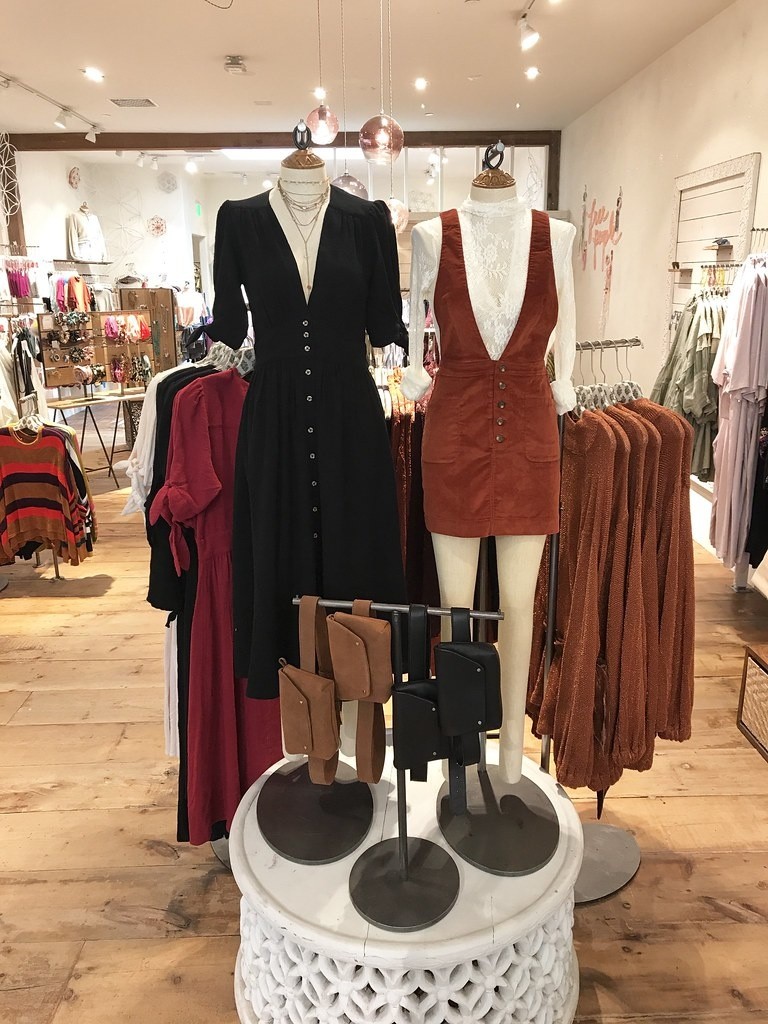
[330,0,368,201]
[307,0,339,146]
[383,0,409,234]
[357,0,404,166]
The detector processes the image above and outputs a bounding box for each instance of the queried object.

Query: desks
[45,386,147,488]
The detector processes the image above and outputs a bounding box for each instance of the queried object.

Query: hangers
[3,243,40,274]
[197,334,254,384]
[374,343,404,371]
[695,263,744,300]
[568,337,645,419]
[748,227,768,256]
[10,304,30,339]
[11,395,43,435]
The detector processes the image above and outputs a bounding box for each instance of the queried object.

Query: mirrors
[664,152,761,505]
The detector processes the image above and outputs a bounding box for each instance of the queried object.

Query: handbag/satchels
[394,603,452,781]
[278,596,343,786]
[433,606,504,765]
[326,597,393,785]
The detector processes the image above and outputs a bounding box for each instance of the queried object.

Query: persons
[69,201,108,262]
[210,129,409,785]
[411,148,577,786]
[173,278,208,363]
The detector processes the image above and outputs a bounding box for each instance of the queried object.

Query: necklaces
[271,176,332,296]
